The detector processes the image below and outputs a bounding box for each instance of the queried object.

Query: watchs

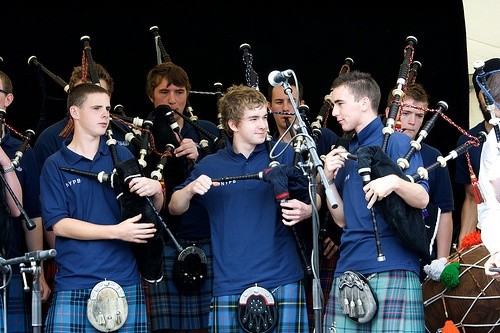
[4,163,15,173]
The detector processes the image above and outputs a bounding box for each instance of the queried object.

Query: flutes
[0,108,36,231]
[28,25,314,275]
[330,35,488,261]
[284,58,353,166]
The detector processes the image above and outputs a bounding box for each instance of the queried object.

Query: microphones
[268,69,292,86]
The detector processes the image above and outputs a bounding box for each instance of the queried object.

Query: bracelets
[328,178,334,184]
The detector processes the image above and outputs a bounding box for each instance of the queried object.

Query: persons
[41,82,149,333]
[452,58,500,284]
[315,72,453,333]
[0,68,33,333]
[169,83,321,333]
[33,65,113,288]
[139,63,228,333]
[266,78,339,333]
